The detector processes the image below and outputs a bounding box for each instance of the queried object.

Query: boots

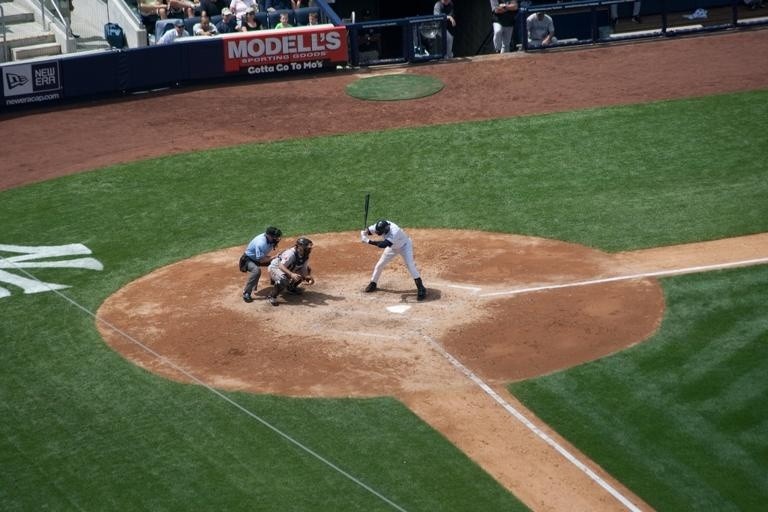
[414,278,426,300]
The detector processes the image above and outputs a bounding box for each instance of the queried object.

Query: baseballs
[308,280,313,284]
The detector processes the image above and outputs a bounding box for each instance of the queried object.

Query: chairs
[137,1,326,50]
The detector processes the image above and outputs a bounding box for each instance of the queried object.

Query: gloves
[360,230,370,244]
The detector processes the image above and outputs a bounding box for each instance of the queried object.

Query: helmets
[266,226,282,238]
[374,219,389,236]
[297,237,313,251]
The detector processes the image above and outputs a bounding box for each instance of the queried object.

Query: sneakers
[286,284,305,293]
[365,282,377,292]
[268,294,277,305]
[242,292,253,303]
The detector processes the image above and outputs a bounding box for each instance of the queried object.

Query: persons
[356,8,383,59]
[361,220,426,301]
[526,11,557,49]
[268,237,315,307]
[433,0,457,59]
[137,0,322,46]
[610,0,643,24]
[490,1,518,53]
[242,227,283,302]
[745,0,767,10]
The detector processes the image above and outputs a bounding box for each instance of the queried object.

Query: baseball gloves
[302,275,314,286]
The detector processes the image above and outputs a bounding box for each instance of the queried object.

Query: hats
[173,19,185,29]
[220,6,233,16]
[245,7,255,13]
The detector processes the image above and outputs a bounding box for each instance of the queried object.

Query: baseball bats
[363,194,371,230]
